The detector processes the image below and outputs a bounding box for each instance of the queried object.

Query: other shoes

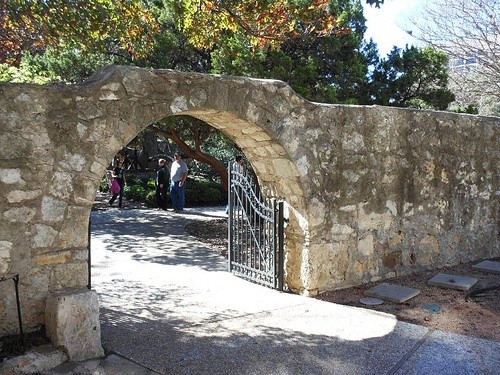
[154,207,167,211]
[172,208,183,214]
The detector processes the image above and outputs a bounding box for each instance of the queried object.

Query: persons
[156,159,170,211]
[170,151,188,213]
[108,169,124,208]
[226,156,246,214]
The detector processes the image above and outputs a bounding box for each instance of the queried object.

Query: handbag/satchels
[110,178,120,194]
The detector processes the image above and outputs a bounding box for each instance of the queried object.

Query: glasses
[119,162,122,163]
